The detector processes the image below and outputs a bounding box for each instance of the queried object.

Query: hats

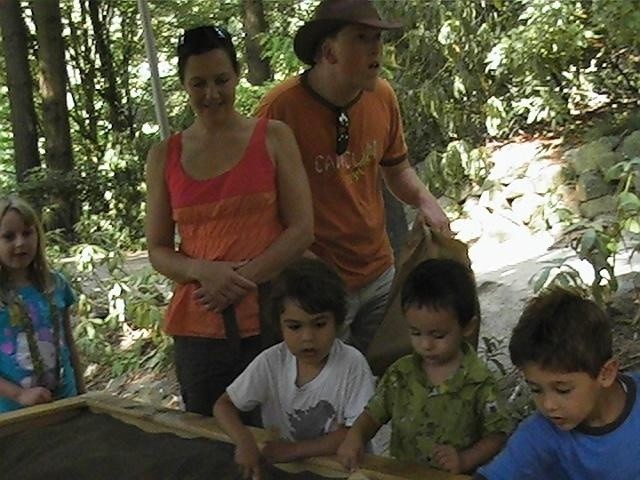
[294,0,404,66]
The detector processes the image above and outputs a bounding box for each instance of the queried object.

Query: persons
[145,28,315,429]
[0,197,86,413]
[462,285,640,480]
[214,257,378,480]
[251,3,455,351]
[333,259,516,480]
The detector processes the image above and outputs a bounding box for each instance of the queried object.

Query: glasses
[177,25,231,55]
[336,107,351,156]
